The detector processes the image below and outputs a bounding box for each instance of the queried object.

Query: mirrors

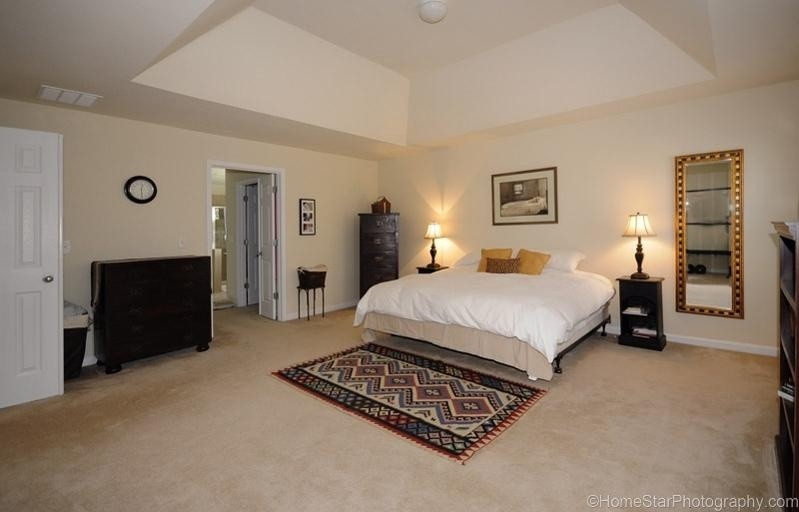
[675,147,745,320]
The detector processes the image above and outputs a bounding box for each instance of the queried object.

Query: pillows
[517,246,586,273]
[485,257,519,275]
[453,246,513,267]
[516,249,551,277]
[478,247,512,276]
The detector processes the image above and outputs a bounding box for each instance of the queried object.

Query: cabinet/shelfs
[775,236,798,511]
[90,254,213,375]
[357,213,399,302]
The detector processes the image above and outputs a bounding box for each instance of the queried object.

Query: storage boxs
[296,264,328,288]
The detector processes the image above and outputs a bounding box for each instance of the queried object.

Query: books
[631,326,657,339]
[777,382,795,403]
[622,304,649,316]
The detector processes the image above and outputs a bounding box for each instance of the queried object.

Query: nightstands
[416,266,448,274]
[616,275,667,352]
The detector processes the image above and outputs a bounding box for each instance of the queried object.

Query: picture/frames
[621,213,658,280]
[491,166,558,227]
[300,198,317,237]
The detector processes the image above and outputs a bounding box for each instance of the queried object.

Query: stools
[298,286,325,322]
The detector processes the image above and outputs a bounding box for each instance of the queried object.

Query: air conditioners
[35,85,102,109]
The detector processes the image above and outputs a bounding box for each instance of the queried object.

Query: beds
[352,245,616,381]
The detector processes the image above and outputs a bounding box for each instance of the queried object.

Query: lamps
[423,223,444,268]
[417,0,448,24]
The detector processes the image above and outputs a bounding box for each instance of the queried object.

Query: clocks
[123,175,158,204]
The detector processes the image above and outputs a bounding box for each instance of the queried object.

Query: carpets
[266,340,551,466]
